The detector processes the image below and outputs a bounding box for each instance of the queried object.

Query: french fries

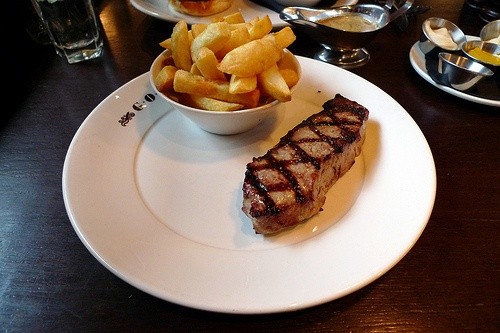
[154,12,299,113]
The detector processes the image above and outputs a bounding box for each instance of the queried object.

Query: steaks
[240,92,370,235]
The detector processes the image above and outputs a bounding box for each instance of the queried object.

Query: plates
[409,35,500,107]
[130,0,291,28]
[62,55,437,314]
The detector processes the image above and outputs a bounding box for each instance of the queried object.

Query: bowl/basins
[150,49,302,136]
[461,40,500,80]
[480,19,500,46]
[438,52,494,91]
[420,17,465,60]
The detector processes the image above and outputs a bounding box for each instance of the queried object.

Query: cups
[32,0,104,64]
